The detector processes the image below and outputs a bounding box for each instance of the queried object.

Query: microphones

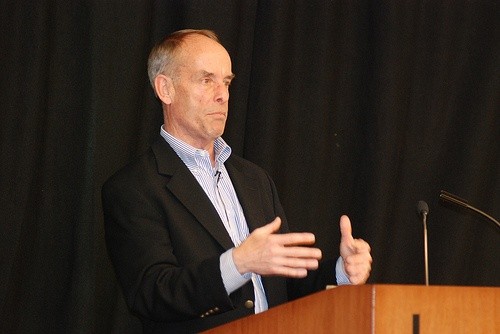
[438,192,500,232]
[416,200,430,286]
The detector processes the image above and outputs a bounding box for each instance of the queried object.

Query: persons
[101,29,375,334]
